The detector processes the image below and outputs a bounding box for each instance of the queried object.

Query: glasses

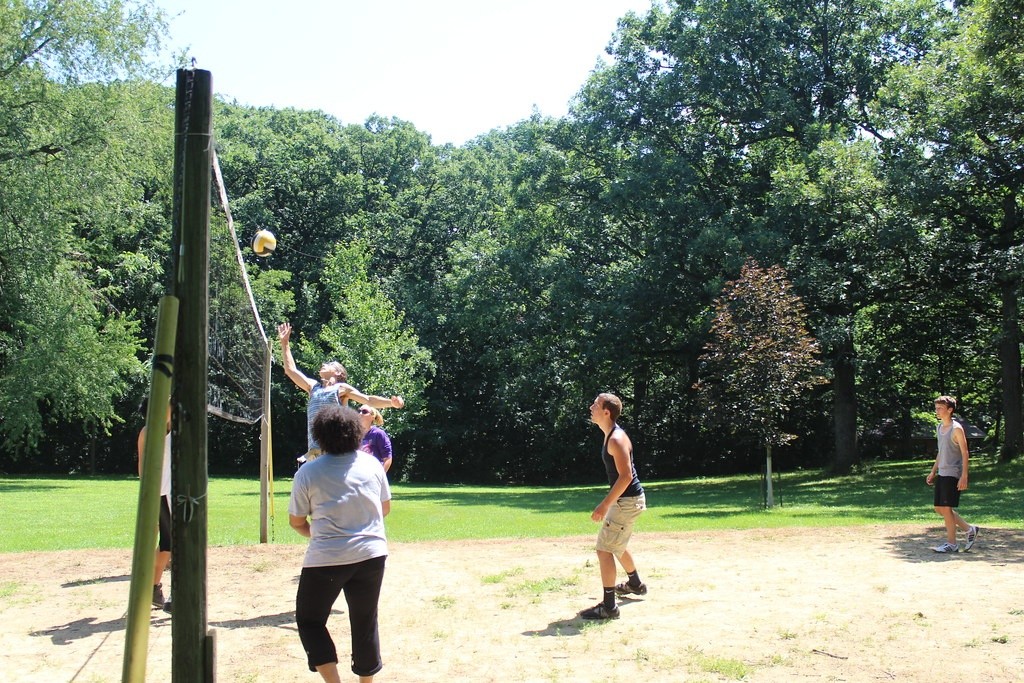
[357,408,373,415]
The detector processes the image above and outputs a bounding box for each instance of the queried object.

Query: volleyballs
[249,230,277,259]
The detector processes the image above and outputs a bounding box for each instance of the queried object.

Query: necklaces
[320,379,334,385]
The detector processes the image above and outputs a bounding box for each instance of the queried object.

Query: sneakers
[614,580,647,595]
[964,526,979,552]
[931,542,958,552]
[580,601,620,620]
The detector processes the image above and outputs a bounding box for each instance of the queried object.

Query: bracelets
[283,347,291,349]
[931,469,936,473]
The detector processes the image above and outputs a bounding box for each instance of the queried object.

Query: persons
[278,321,404,462]
[580,393,648,619]
[926,395,979,554]
[288,405,393,683]
[137,396,172,608]
[355,404,392,473]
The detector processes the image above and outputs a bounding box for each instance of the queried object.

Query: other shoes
[152,582,172,615]
